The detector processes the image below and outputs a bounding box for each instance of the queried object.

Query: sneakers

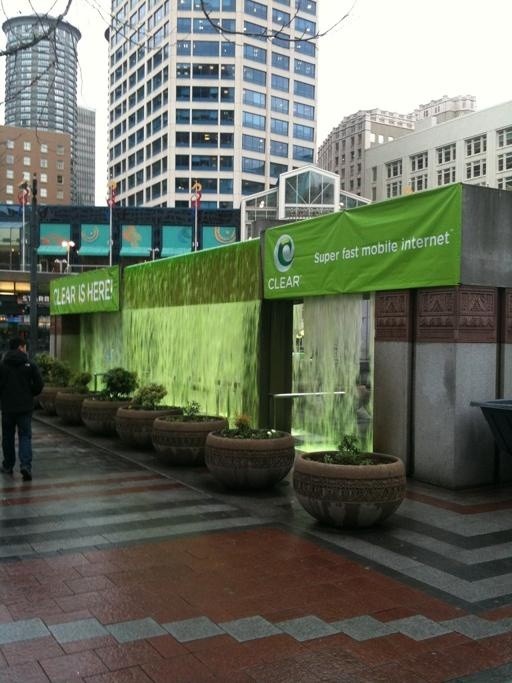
[20,467,32,482]
[0,466,13,474]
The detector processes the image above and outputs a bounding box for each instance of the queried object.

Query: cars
[5,299,50,328]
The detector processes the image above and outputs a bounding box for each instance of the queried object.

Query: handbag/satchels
[28,373,44,397]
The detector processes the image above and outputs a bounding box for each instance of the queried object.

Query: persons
[1,333,45,483]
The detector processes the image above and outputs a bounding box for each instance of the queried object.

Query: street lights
[147,246,160,259]
[62,239,76,273]
[19,180,30,272]
[54,258,67,271]
